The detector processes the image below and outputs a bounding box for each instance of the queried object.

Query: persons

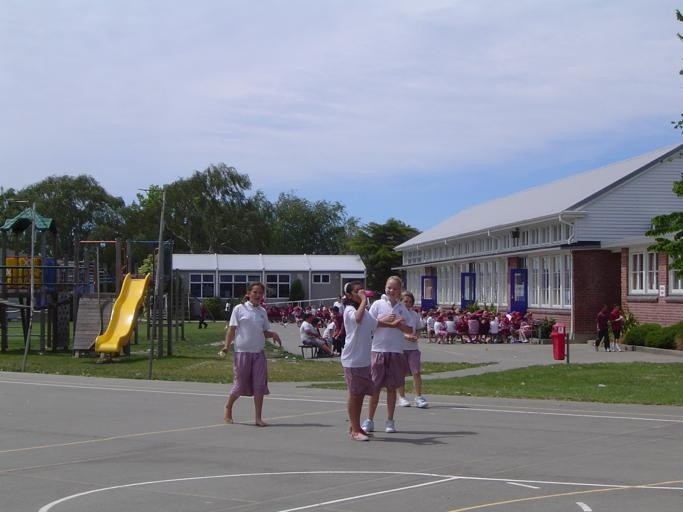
[360,277,413,433]
[610,301,626,350]
[217,282,282,425]
[198,303,206,329]
[262,295,346,357]
[593,303,610,353]
[224,296,231,330]
[340,280,404,442]
[398,291,428,408]
[412,302,534,344]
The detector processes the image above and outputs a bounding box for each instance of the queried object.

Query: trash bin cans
[550,323,566,360]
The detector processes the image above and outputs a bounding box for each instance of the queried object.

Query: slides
[94,272,151,352]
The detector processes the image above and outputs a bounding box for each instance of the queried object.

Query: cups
[364,290,376,297]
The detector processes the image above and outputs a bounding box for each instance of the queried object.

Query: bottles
[618,315,624,321]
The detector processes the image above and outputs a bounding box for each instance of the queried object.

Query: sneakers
[415,396,429,407]
[347,430,374,442]
[361,418,375,433]
[384,419,396,434]
[398,396,411,408]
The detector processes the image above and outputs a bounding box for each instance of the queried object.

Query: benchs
[446,334,510,344]
[298,345,319,359]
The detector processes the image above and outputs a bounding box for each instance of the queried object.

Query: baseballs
[218,351,226,359]
